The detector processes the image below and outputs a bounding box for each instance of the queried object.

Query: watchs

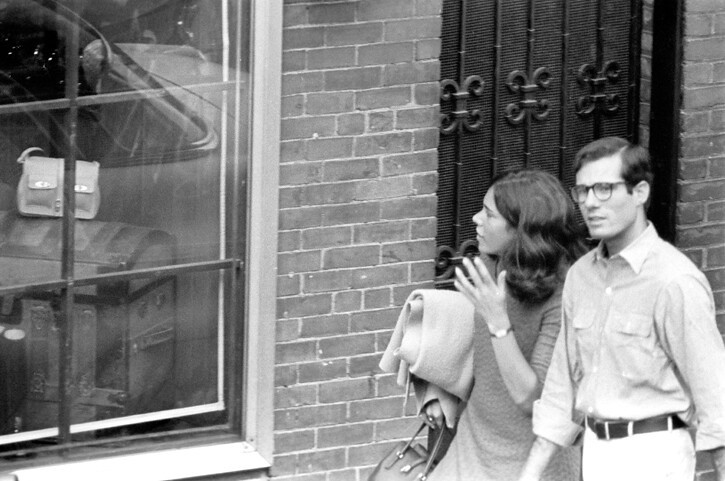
[490,324,515,338]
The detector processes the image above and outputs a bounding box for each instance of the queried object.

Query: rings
[427,417,433,422]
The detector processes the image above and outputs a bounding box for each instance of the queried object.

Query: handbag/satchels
[367,415,446,481]
[16,156,101,219]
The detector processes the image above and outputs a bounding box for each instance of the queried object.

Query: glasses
[569,182,638,204]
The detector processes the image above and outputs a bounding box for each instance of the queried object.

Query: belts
[587,413,690,440]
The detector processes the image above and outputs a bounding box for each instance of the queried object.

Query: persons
[418,171,596,481]
[521,136,725,481]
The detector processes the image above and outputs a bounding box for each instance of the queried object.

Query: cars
[0,0,251,443]
